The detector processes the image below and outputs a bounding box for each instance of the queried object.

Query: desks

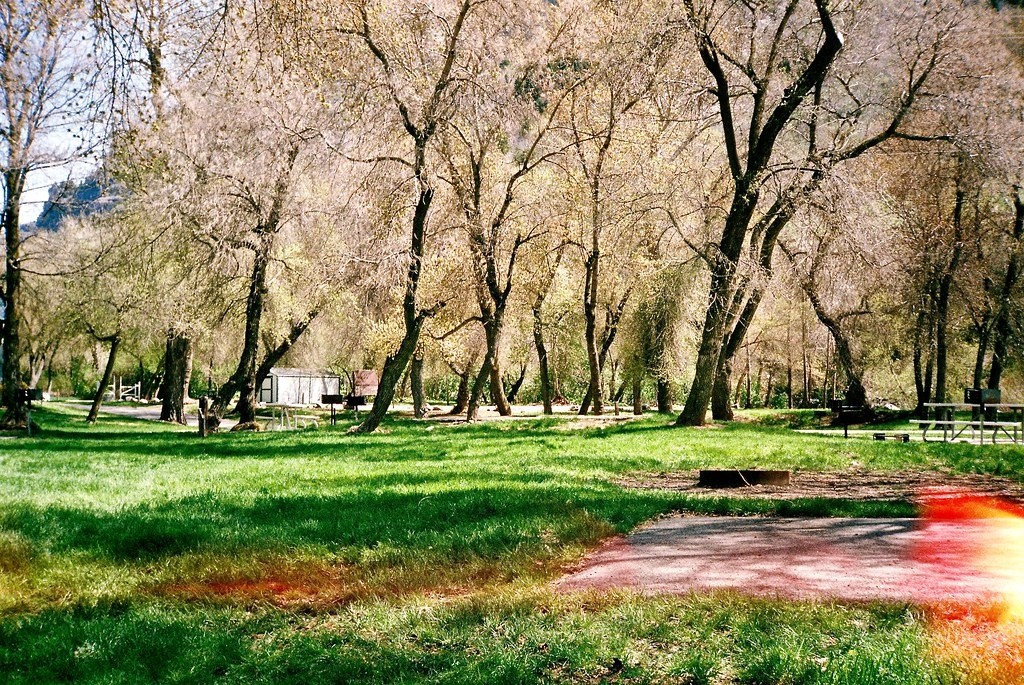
[265,404,317,430]
[922,403,1024,443]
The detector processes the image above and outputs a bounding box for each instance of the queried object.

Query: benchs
[255,416,278,430]
[908,419,1021,444]
[293,415,321,429]
